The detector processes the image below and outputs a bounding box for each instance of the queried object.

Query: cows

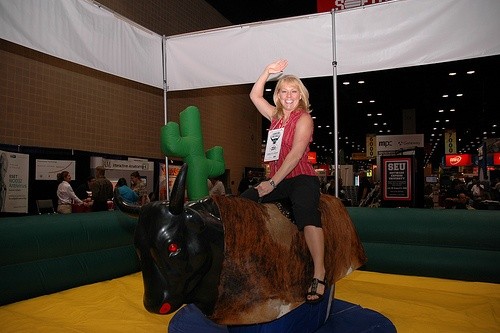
[114,163,368,333]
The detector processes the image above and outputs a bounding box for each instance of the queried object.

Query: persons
[206,177,225,196]
[240,168,500,211]
[56,171,86,214]
[249,59,329,301]
[87,167,151,213]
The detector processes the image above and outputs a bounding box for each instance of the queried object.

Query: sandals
[306,274,328,302]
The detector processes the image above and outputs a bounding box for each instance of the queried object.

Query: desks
[70,199,114,212]
[445,198,500,211]
[345,207,500,284]
[0,211,142,307]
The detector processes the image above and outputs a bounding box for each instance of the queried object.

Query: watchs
[269,179,276,188]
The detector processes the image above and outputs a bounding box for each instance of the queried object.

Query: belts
[58,203,71,205]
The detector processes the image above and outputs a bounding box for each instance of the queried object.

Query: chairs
[36,200,55,215]
